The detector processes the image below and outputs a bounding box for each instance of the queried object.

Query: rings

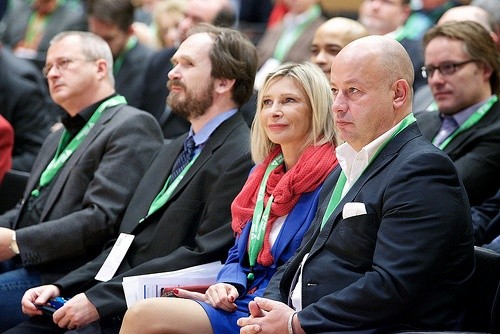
[68,324,72,329]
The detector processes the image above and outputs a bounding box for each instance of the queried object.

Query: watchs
[9,231,20,254]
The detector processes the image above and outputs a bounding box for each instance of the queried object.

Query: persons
[0,0,500,334]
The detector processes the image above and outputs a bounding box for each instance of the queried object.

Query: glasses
[421,59,477,78]
[41,57,82,78]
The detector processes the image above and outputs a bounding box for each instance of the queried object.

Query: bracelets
[288,311,298,334]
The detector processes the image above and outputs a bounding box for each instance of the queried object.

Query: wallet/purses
[161,284,211,297]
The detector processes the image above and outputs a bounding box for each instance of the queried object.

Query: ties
[166,134,198,191]
